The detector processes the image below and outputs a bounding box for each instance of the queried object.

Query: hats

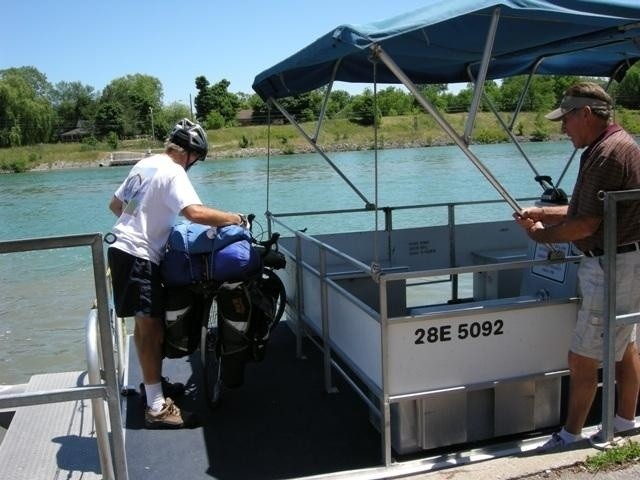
[544,94,615,122]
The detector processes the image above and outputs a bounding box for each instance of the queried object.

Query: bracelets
[235,212,248,229]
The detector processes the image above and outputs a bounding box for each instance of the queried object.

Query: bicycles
[197,214,287,408]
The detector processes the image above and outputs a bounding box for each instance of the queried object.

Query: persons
[511,81,639,452]
[103,115,250,428]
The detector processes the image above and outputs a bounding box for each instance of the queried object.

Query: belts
[583,243,640,259]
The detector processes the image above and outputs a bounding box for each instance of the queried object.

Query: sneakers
[139,375,184,403]
[536,433,564,451]
[144,396,202,430]
[590,427,619,440]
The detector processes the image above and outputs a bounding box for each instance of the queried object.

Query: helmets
[167,117,210,162]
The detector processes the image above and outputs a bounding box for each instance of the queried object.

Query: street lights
[149,106,155,140]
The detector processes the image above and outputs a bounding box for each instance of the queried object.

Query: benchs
[470,247,530,301]
[311,260,409,320]
